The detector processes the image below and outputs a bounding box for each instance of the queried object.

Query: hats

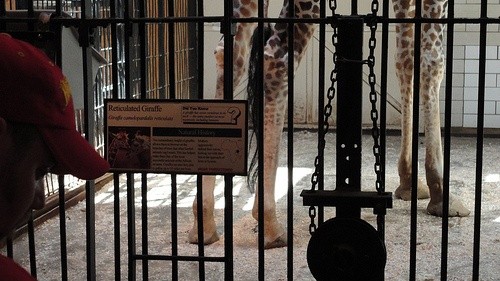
[0,33,110,180]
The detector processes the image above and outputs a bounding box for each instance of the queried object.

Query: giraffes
[186,0,471,250]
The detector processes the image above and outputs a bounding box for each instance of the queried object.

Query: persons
[0,34,112,281]
